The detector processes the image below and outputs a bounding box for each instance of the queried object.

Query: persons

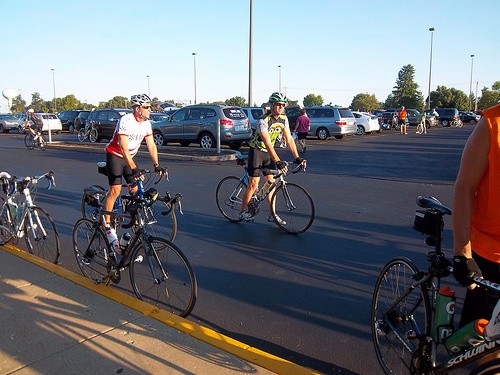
[103,93,166,248]
[380,116,390,125]
[416,109,427,134]
[397,106,408,135]
[294,108,310,154]
[452,104,500,330]
[432,108,440,126]
[237,92,306,225]
[25,109,44,147]
[391,115,397,129]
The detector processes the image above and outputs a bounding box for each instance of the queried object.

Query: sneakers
[239,211,254,221]
[267,213,286,225]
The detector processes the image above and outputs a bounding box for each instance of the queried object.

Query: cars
[243,105,303,135]
[0,107,165,142]
[351,111,381,136]
[376,108,481,128]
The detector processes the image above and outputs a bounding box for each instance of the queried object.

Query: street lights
[278,65,281,92]
[51,69,56,113]
[427,28,434,110]
[147,75,150,97]
[470,55,474,111]
[192,53,197,104]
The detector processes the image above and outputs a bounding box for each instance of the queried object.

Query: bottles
[260,180,272,195]
[444,318,490,356]
[431,286,457,343]
[16,203,24,222]
[106,227,120,253]
[117,232,131,254]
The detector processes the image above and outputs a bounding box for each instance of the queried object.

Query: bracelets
[273,156,279,161]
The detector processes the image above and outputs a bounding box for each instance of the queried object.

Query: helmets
[130,94,152,108]
[268,92,287,105]
[28,109,35,112]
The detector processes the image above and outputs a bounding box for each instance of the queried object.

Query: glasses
[141,106,151,110]
[275,103,286,108]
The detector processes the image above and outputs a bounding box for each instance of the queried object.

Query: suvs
[152,104,253,150]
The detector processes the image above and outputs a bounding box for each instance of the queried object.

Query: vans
[303,106,357,139]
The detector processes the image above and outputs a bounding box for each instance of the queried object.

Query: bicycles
[78,119,101,143]
[216,160,315,235]
[82,161,177,251]
[370,193,500,375]
[24,125,47,151]
[0,170,61,265]
[72,184,197,319]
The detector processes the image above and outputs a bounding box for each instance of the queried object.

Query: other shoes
[33,135,38,140]
